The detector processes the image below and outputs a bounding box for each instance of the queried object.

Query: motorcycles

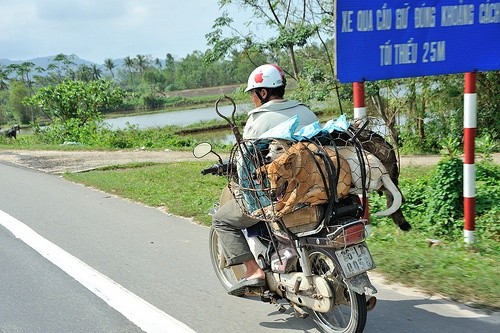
[192,140,379,333]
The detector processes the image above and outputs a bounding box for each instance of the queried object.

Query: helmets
[243,61,287,92]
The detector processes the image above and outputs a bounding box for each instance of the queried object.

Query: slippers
[227,277,267,296]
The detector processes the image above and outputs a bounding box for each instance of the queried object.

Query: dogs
[249,133,413,232]
[265,138,401,218]
[269,143,351,218]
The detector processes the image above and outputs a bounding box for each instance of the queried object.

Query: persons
[210,63,320,296]
